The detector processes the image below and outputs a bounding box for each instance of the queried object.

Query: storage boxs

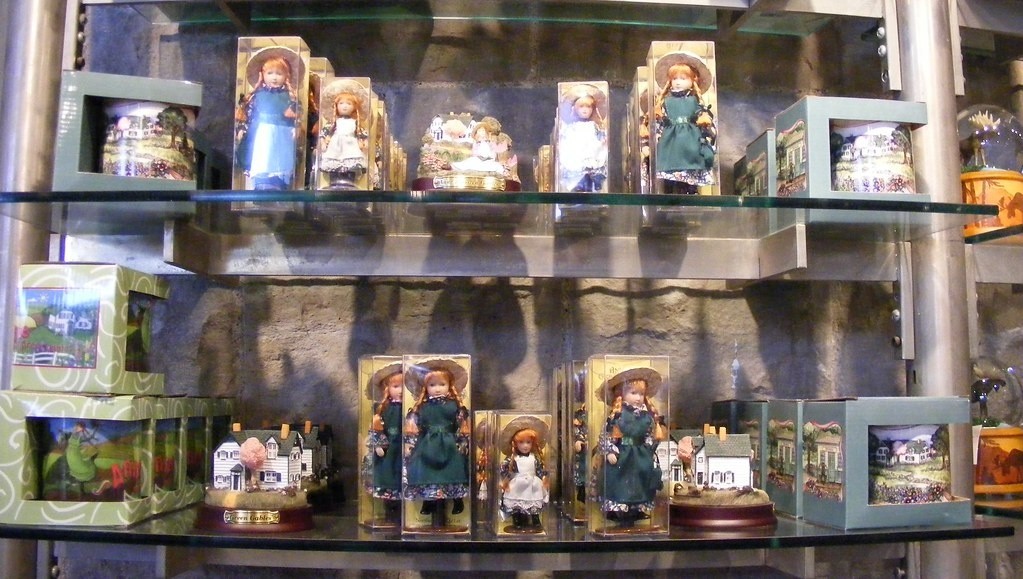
[0,33,978,540]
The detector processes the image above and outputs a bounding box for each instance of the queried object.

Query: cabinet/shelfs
[0,182,1011,554]
